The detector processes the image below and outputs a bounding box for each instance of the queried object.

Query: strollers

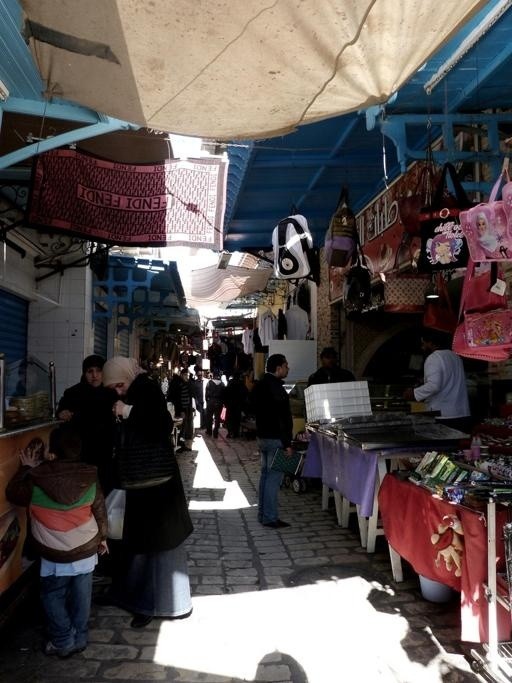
[280,430,313,495]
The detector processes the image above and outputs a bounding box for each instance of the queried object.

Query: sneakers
[46,642,76,660]
[131,613,153,629]
[264,519,291,530]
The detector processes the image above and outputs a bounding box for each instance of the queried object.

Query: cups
[462,444,481,462]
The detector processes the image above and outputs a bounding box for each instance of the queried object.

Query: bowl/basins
[420,573,450,604]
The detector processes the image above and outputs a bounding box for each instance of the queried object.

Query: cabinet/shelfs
[469,490,512,683]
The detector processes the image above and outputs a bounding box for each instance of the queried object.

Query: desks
[303,419,471,552]
[378,459,510,645]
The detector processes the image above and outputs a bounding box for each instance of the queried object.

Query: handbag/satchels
[271,214,321,287]
[394,182,512,363]
[325,205,385,315]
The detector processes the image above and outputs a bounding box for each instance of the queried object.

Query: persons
[168,365,253,454]
[306,346,356,387]
[55,355,120,497]
[93,356,194,628]
[5,422,112,660]
[403,326,475,436]
[249,354,293,529]
